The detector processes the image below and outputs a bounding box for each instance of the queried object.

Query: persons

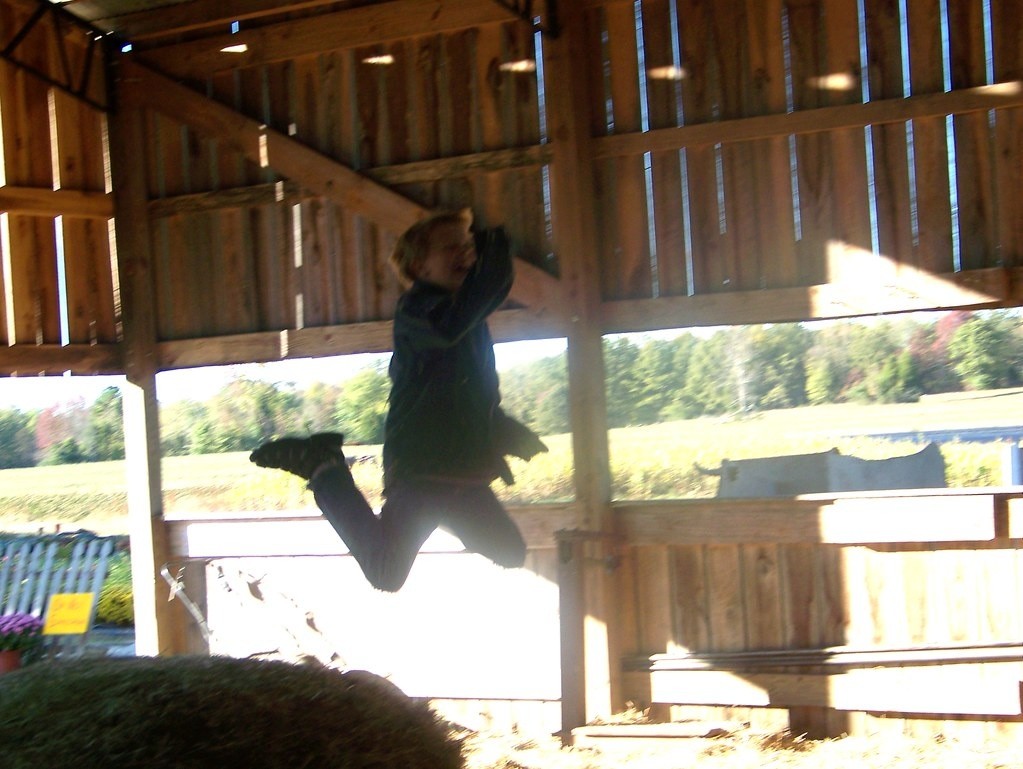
[252,209,547,590]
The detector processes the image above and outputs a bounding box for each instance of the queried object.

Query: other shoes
[250,432,345,480]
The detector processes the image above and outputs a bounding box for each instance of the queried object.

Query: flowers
[0,612,46,651]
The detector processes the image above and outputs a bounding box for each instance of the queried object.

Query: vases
[0,651,20,674]
